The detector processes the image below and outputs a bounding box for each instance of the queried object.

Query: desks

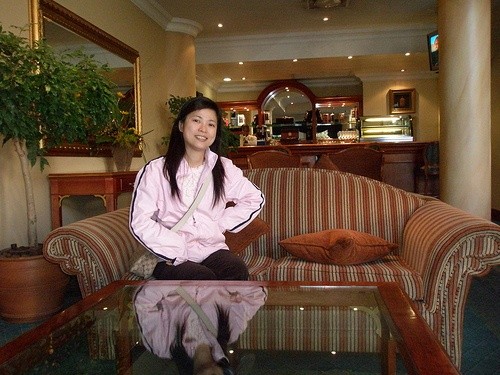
[0,279,461,375]
[48,171,137,233]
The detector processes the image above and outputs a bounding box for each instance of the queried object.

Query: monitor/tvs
[427,29,439,71]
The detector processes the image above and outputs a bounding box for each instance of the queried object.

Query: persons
[134,281,268,375]
[128,96,266,281]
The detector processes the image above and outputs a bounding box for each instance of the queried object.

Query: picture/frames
[389,89,416,114]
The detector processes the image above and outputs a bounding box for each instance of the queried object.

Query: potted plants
[0,21,143,322]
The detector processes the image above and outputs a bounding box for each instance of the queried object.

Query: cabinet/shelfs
[226,143,423,195]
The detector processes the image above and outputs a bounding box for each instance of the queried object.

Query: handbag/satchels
[126,244,158,278]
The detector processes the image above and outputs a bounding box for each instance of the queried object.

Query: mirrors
[28,0,142,156]
[216,80,361,142]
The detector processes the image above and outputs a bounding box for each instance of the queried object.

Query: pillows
[221,203,268,253]
[280,229,396,264]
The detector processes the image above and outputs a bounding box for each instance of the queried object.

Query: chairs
[247,151,300,169]
[327,147,384,181]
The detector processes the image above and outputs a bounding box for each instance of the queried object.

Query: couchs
[42,168,500,369]
